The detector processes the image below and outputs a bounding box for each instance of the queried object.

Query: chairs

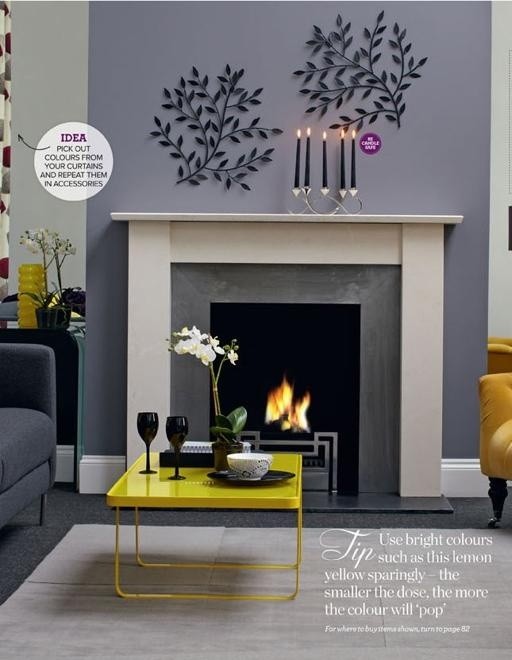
[478,372,512,529]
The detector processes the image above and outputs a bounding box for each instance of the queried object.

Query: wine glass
[136,411,159,474]
[166,416,189,480]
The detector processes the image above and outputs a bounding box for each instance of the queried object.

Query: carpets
[0,524,512,660]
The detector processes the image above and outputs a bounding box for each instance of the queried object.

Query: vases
[34,306,71,330]
[211,442,243,472]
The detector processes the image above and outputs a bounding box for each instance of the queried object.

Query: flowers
[18,224,82,309]
[164,324,248,446]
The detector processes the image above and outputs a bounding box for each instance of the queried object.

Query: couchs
[0,344,58,532]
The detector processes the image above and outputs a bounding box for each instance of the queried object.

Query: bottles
[16,262,48,329]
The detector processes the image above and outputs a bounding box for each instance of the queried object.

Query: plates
[206,468,296,487]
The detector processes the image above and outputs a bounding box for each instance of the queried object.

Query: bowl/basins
[226,452,274,481]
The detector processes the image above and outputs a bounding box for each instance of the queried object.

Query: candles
[293,126,358,188]
